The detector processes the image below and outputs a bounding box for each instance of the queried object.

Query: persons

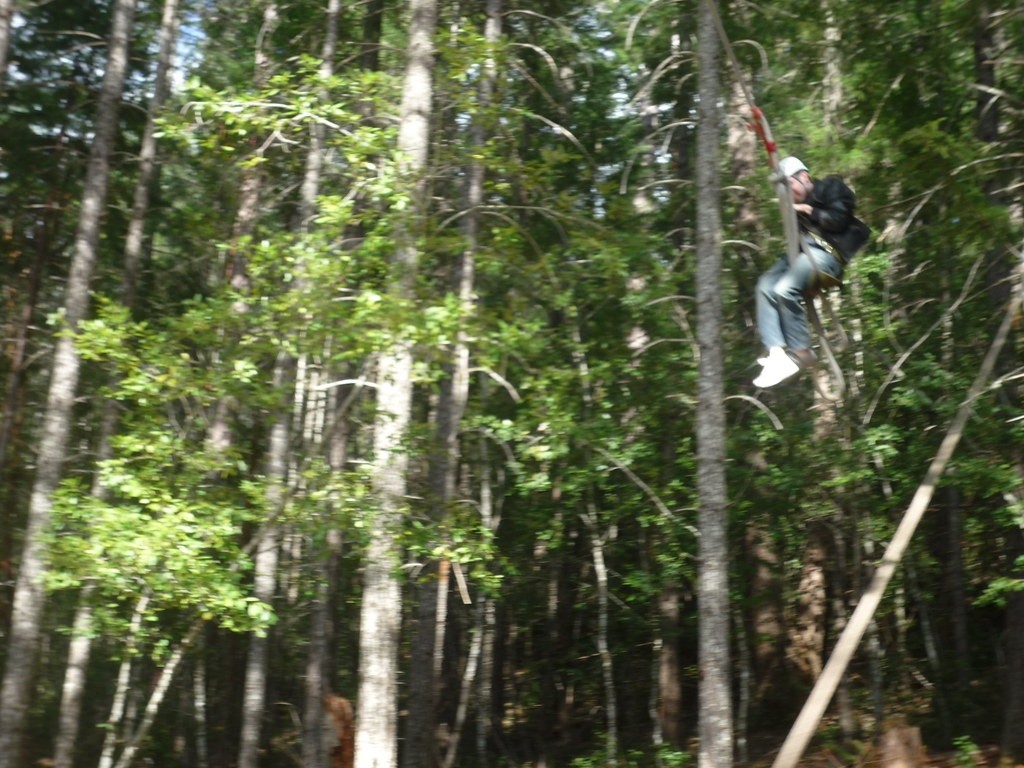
[323,679,354,768]
[752,156,856,389]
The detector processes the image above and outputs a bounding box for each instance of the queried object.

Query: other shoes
[752,355,799,387]
[757,349,819,370]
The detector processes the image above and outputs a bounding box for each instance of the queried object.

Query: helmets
[773,156,809,181]
